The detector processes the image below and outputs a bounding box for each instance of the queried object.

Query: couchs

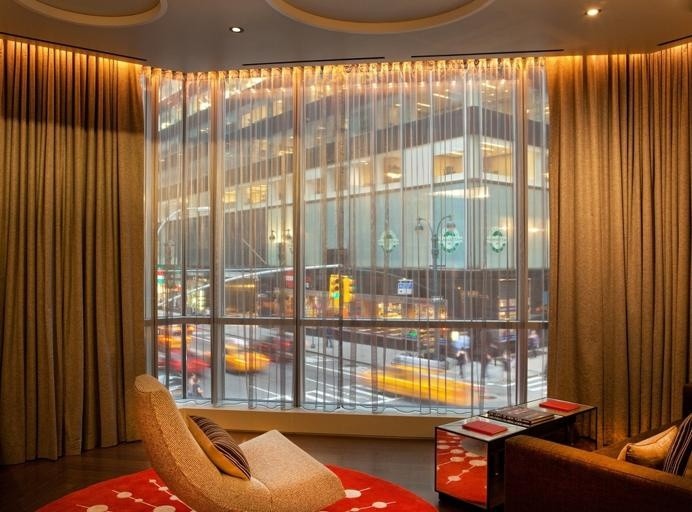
[504,384,691,511]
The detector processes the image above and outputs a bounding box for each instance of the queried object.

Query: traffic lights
[330,274,354,303]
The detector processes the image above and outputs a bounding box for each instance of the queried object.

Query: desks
[435,396,598,512]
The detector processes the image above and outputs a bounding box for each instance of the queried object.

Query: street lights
[269,229,292,269]
[414,215,456,360]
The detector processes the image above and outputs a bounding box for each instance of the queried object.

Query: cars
[158,349,209,374]
[203,344,271,372]
[251,334,294,362]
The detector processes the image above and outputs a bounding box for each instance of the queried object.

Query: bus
[157,324,194,351]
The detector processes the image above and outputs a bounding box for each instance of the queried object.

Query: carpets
[36,465,439,511]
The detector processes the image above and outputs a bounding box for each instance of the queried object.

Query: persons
[456,346,468,377]
[502,350,512,381]
[485,350,493,376]
[326,326,335,348]
[192,378,203,399]
[189,371,200,388]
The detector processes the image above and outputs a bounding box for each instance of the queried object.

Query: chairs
[133,373,346,511]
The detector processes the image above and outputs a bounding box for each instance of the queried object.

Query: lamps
[269,231,276,240]
[284,229,292,239]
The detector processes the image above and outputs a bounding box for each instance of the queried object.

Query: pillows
[617,425,678,470]
[185,414,251,481]
[662,412,692,477]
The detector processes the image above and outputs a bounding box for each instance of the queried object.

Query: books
[539,400,581,412]
[462,420,507,436]
[487,405,555,428]
